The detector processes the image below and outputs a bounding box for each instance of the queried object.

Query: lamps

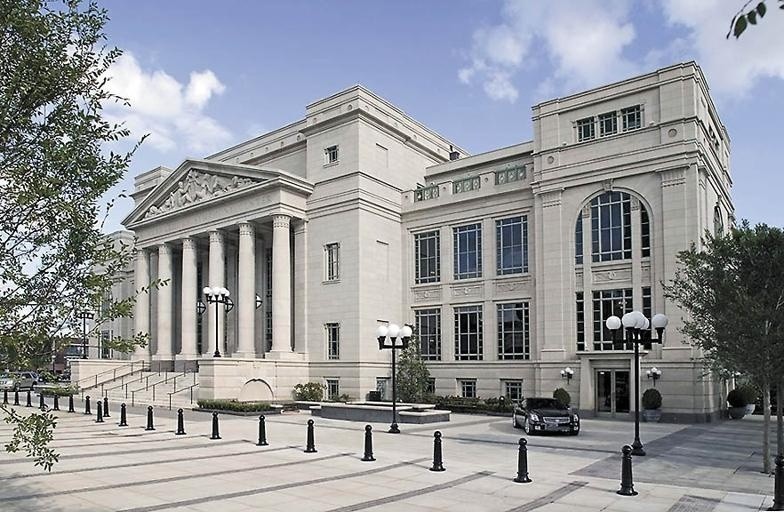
[561,366,573,384]
[196,299,206,316]
[646,367,661,387]
[256,293,262,309]
[225,297,234,313]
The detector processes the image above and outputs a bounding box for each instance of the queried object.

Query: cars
[509,396,582,437]
[56,374,70,381]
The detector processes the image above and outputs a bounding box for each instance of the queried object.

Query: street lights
[373,322,413,433]
[604,309,669,458]
[51,355,56,376]
[203,286,231,357]
[71,302,97,359]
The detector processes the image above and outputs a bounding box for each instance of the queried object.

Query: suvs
[0,371,38,392]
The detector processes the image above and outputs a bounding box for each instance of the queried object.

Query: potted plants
[726,390,747,420]
[552,388,572,413]
[642,388,662,422]
[740,384,756,416]
